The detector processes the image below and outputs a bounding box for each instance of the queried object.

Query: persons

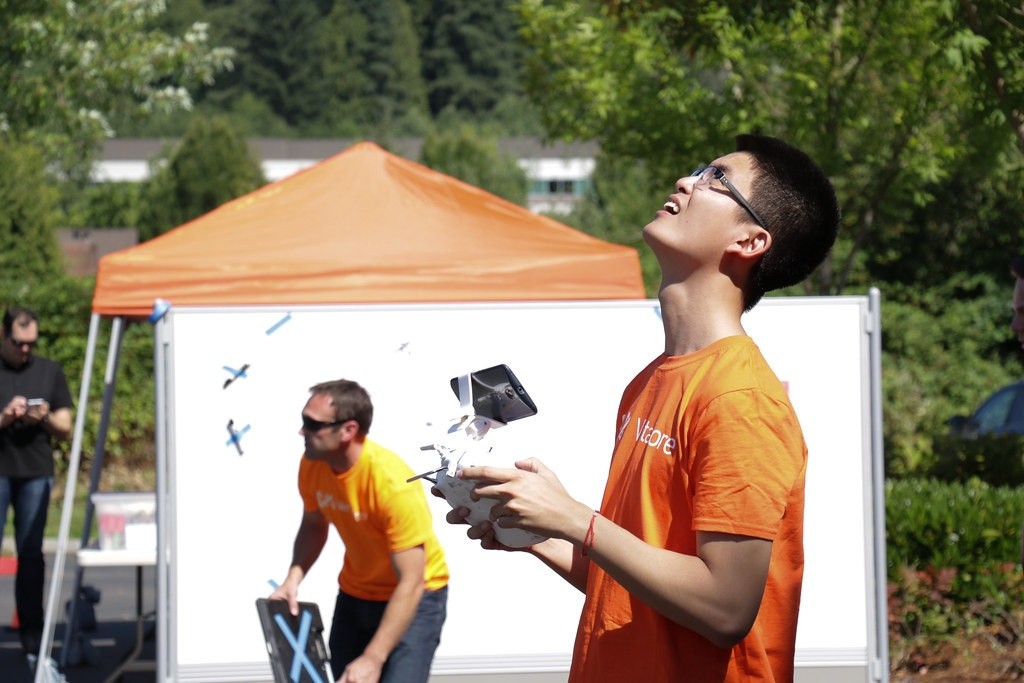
[1011,258,1024,349]
[431,135,841,683]
[265,379,451,683]
[0,307,74,683]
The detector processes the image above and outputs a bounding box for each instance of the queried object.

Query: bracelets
[581,511,597,559]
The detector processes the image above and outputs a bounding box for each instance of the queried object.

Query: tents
[29,142,649,683]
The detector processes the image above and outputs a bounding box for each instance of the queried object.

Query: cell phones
[451,364,538,423]
[26,399,44,410]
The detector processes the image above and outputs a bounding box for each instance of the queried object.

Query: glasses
[6,335,37,348]
[689,165,769,270]
[301,414,350,433]
